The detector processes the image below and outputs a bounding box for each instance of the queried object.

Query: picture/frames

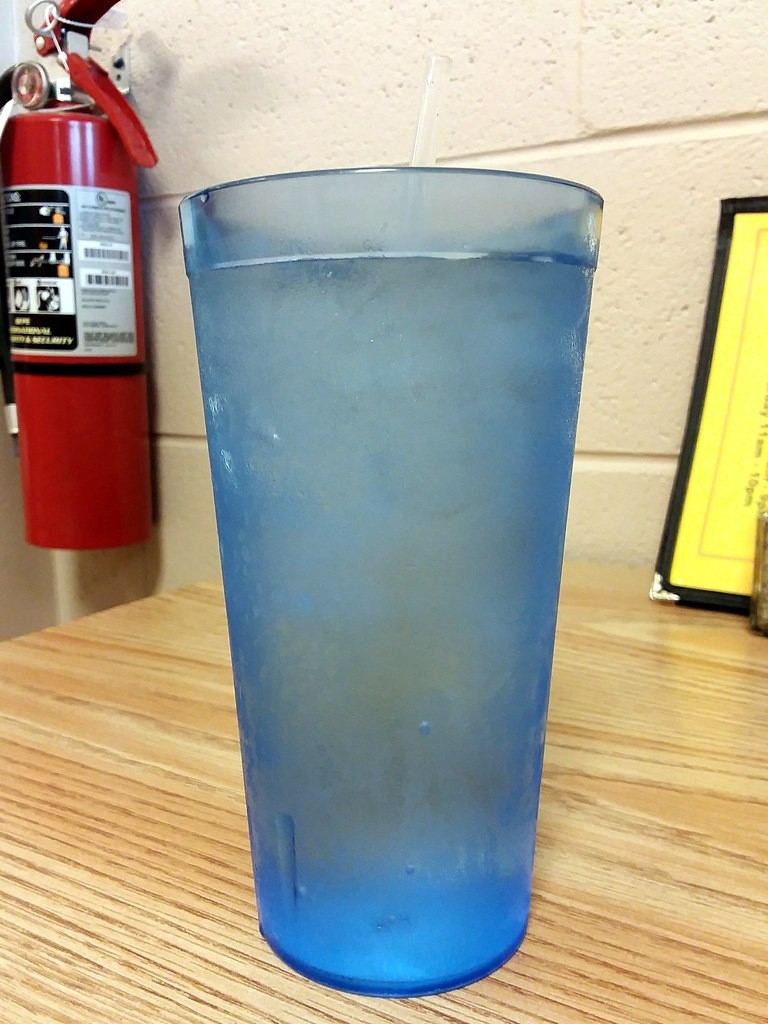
[649,196,767,611]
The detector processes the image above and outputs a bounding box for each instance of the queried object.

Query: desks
[0,573,768,1024]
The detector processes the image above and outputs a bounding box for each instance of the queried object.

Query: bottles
[750,511,768,634]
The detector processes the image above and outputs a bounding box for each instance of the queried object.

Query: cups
[177,168,605,999]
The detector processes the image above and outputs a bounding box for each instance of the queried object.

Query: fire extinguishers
[1,1,158,549]
